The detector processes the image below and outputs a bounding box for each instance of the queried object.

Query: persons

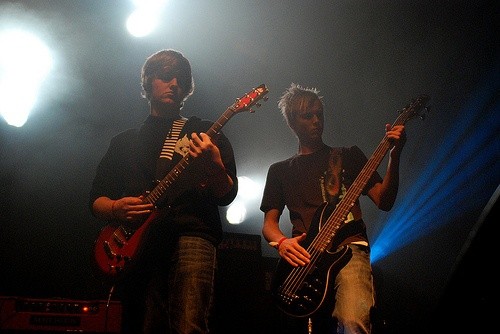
[89,49,238,334]
[261,81,407,334]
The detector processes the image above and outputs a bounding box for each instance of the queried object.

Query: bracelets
[276,237,288,251]
[106,201,115,221]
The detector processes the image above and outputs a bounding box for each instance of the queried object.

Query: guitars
[270,91,436,319]
[91,83,270,277]
